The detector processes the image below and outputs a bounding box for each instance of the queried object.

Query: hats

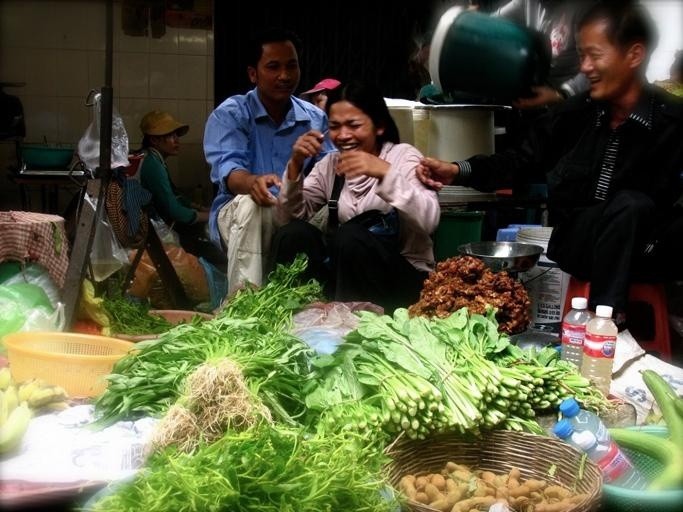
[299,79,341,101]
[140,109,190,137]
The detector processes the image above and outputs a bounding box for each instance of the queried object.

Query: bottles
[579,304,618,398]
[576,429,650,492]
[561,296,593,373]
[552,419,583,454]
[559,398,614,449]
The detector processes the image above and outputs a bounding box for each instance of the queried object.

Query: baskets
[602,424,683,511]
[1,330,141,399]
[379,429,603,512]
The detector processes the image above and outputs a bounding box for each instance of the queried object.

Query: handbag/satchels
[325,210,401,293]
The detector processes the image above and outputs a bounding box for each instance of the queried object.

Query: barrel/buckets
[424,109,495,162]
[414,107,429,156]
[429,5,551,107]
[515,227,572,338]
[389,107,416,147]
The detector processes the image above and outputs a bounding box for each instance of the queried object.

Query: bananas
[0,367,70,454]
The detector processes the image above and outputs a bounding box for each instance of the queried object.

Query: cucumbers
[605,368,683,491]
